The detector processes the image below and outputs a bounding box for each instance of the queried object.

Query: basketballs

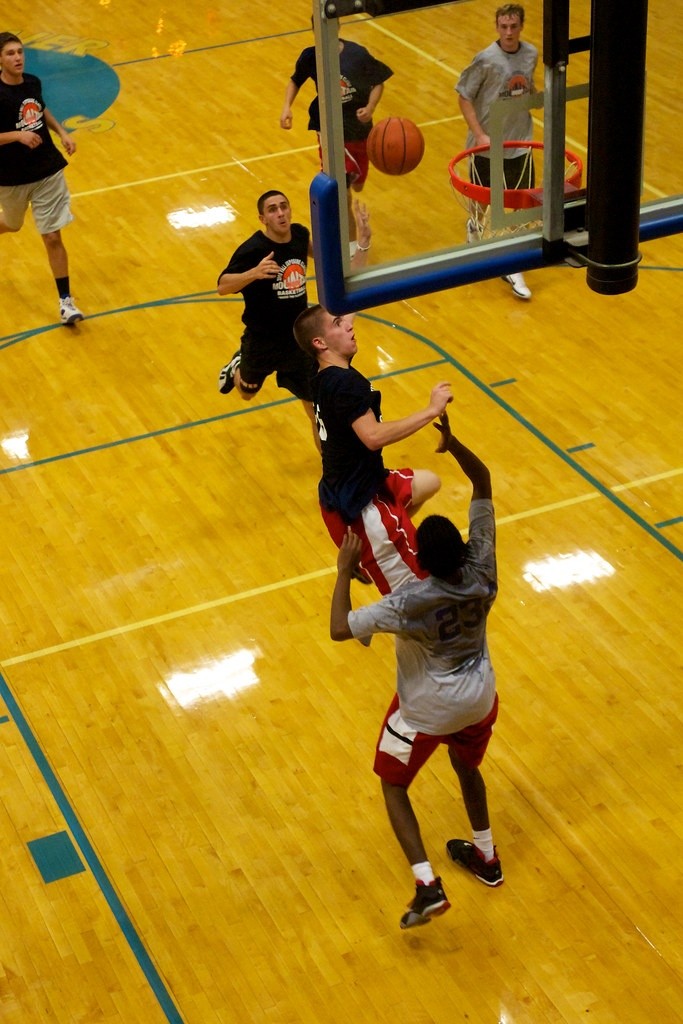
[366,116,427,177]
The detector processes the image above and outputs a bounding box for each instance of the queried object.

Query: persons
[293,198,449,598]
[279,12,394,259]
[456,5,538,300]
[331,407,499,929]
[0,32,83,326]
[217,189,325,457]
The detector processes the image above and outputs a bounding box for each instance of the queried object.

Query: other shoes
[350,566,374,584]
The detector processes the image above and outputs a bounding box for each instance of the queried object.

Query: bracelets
[356,242,371,252]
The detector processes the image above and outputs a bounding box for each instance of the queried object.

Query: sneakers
[502,274,531,298]
[219,350,242,393]
[445,839,505,887]
[401,876,451,927]
[466,218,485,244]
[59,297,83,325]
